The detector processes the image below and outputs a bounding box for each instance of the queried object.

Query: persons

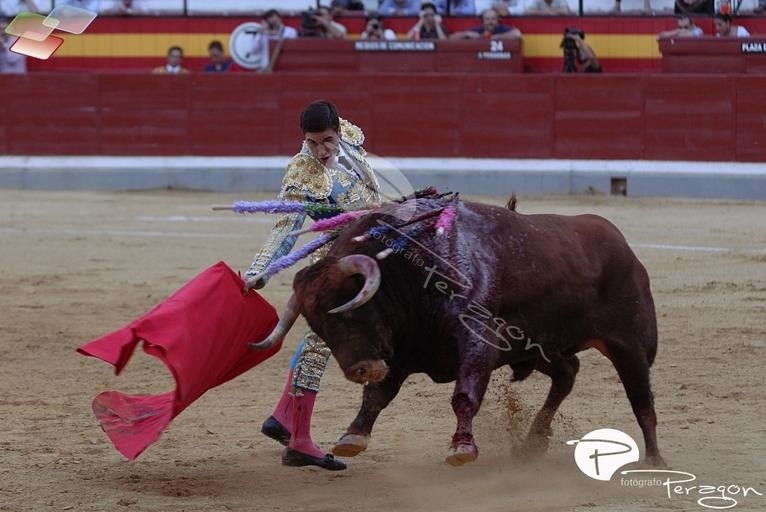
[448,10,523,40]
[713,12,750,37]
[656,10,707,37]
[204,39,235,73]
[152,45,193,74]
[407,3,452,41]
[299,7,350,43]
[246,8,303,74]
[557,22,606,73]
[360,18,397,41]
[236,98,382,473]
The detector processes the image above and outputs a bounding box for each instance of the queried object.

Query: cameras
[267,21,277,30]
[372,23,379,30]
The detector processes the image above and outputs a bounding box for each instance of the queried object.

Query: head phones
[479,9,504,23]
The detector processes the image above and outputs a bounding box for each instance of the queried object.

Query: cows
[246,189,667,471]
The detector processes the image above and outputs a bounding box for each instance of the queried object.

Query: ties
[338,156,364,181]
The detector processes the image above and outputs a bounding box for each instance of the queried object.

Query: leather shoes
[282,447,346,470]
[260,416,290,446]
